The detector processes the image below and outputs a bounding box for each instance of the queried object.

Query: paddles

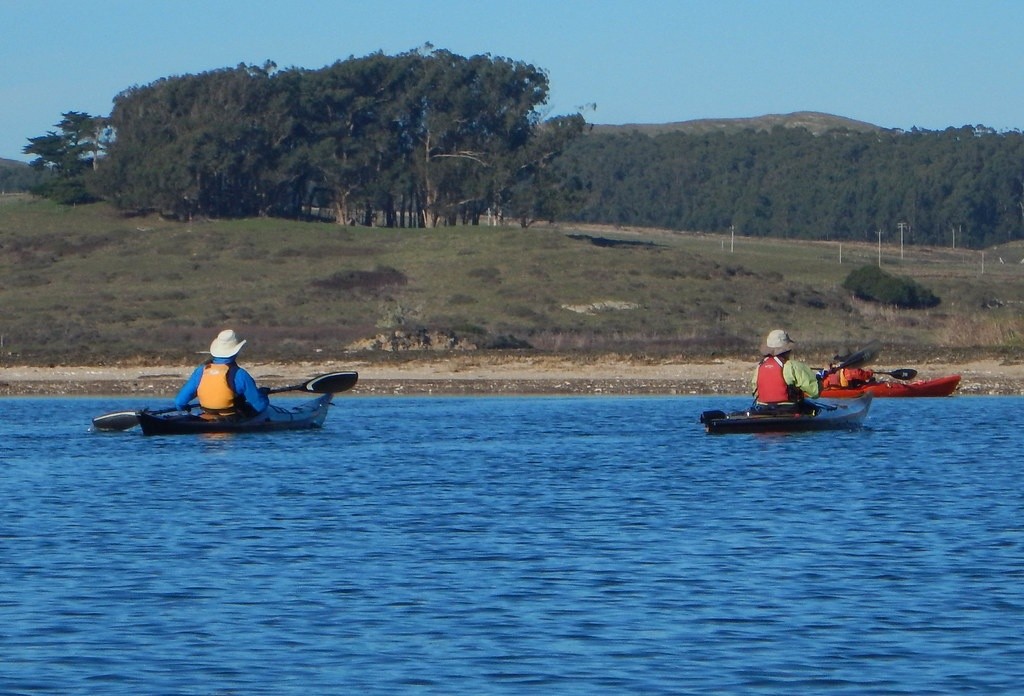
[91,370,359,432]
[810,367,918,380]
[700,342,880,423]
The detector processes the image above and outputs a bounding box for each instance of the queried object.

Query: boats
[137,389,334,436]
[797,375,963,399]
[699,390,873,436]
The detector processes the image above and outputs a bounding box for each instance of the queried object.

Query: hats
[834,345,853,361]
[759,328,796,357]
[209,330,247,357]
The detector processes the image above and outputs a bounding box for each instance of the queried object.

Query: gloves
[259,387,270,394]
[185,404,191,413]
[816,370,827,380]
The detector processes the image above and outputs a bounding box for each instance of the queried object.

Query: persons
[174,330,270,423]
[751,330,824,415]
[816,346,873,390]
